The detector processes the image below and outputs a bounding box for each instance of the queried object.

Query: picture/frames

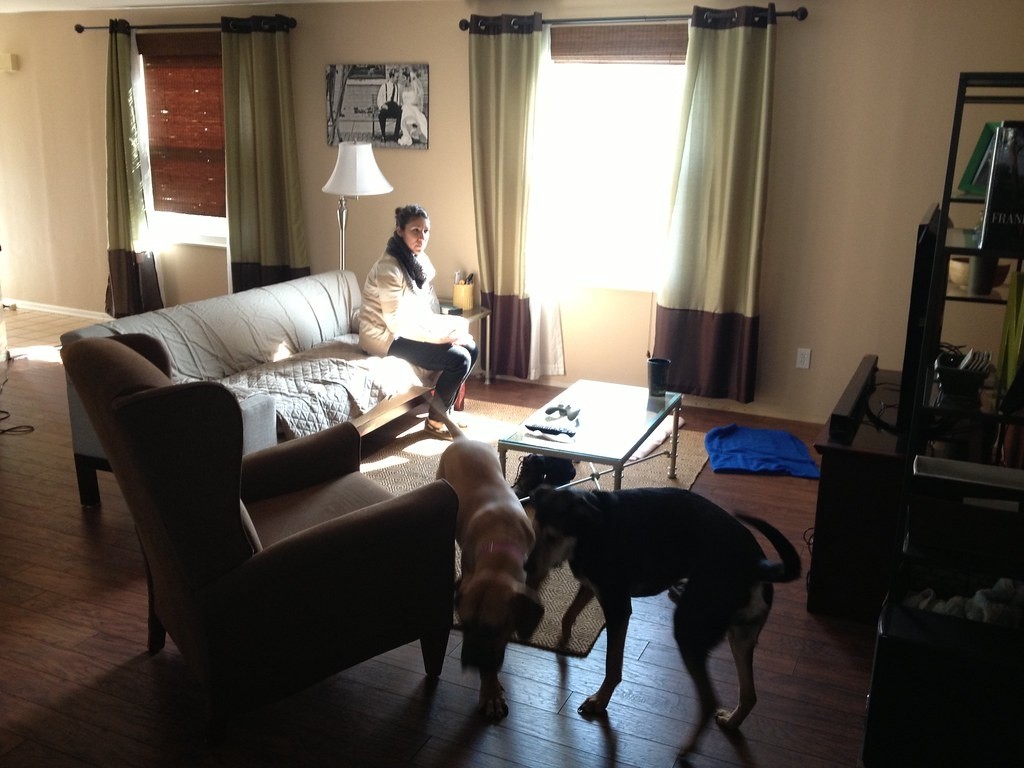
[958,122,1001,197]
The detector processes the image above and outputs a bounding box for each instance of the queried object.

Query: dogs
[410,382,538,723]
[520,478,805,764]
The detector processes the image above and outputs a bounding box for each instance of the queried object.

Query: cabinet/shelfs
[861,456,1024,768]
[898,71,1024,470]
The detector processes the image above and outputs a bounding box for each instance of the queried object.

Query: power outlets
[797,347,811,369]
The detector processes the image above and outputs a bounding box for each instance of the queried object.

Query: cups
[647,357,671,397]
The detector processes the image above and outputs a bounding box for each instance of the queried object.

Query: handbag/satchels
[511,453,578,503]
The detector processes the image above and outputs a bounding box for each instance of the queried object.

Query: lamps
[322,139,393,270]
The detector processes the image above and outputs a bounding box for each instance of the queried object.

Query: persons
[376,65,428,146]
[358,203,480,441]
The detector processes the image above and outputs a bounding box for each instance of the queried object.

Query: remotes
[525,424,575,438]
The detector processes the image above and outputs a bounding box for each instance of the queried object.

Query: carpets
[360,396,709,655]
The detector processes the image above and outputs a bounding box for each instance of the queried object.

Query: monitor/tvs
[896,203,952,456]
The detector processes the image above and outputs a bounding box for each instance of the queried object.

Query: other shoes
[422,414,468,441]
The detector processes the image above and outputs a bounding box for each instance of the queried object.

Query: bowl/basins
[948,258,970,292]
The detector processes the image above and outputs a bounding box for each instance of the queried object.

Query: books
[978,117,1024,253]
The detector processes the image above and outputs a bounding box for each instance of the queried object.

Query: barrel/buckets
[452,284,474,310]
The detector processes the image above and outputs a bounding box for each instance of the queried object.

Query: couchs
[59,271,446,506]
[61,333,459,744]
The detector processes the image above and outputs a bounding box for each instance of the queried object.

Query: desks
[808,354,999,620]
[443,299,491,385]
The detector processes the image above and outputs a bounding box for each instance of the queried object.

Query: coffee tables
[498,379,682,504]
